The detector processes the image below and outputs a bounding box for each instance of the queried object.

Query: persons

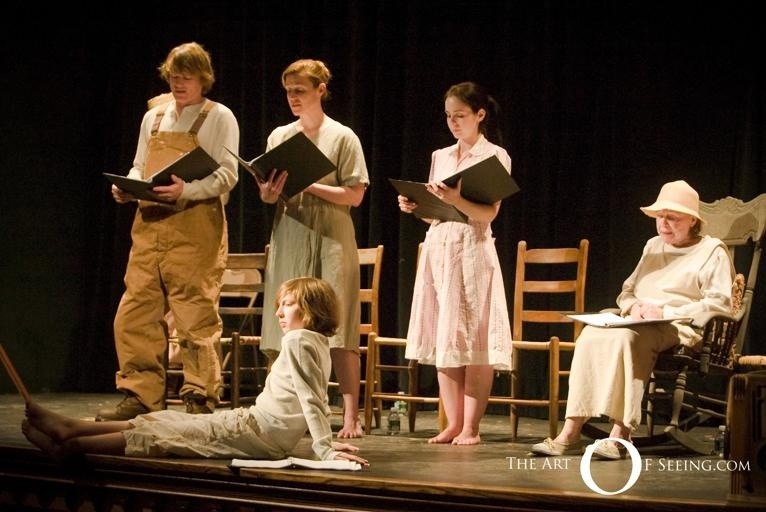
[22,276,370,467]
[94,42,239,421]
[252,60,371,439]
[397,82,513,445]
[531,180,733,461]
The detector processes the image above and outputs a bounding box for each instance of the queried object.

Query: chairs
[364,331,450,436]
[325,244,384,435]
[163,244,271,410]
[484,237,590,440]
[581,190,766,455]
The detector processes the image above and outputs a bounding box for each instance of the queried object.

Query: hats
[639,178,710,229]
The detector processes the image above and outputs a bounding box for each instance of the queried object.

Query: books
[223,131,337,202]
[558,311,692,328]
[231,457,362,472]
[388,154,521,224]
[102,147,221,206]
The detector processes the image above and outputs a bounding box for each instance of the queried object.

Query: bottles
[388,407,400,436]
[393,392,408,416]
[712,425,726,456]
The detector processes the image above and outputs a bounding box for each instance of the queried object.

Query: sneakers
[530,435,585,457]
[593,438,636,461]
[185,399,212,415]
[94,394,152,422]
